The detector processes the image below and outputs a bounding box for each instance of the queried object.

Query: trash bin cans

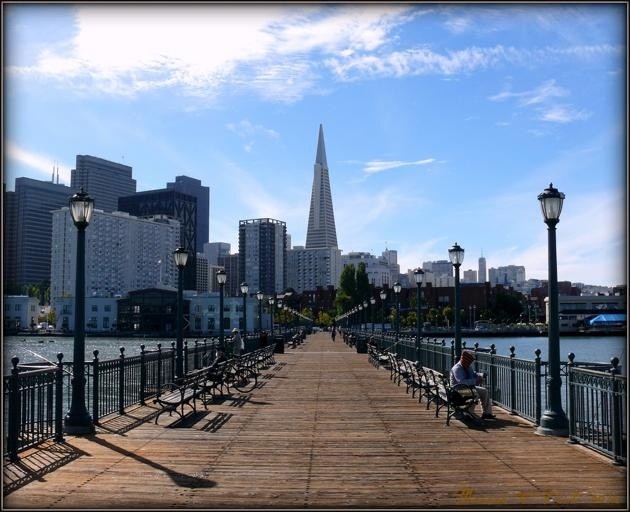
[273,335,284,354]
[357,335,367,353]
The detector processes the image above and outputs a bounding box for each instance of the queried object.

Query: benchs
[152,343,277,425]
[368,341,483,426]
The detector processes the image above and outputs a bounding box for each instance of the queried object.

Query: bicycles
[225,361,258,393]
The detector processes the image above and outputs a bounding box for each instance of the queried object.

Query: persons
[449,349,496,419]
[299,328,303,337]
[206,351,228,395]
[331,323,336,342]
[228,327,242,363]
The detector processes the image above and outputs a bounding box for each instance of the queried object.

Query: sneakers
[482,414,496,419]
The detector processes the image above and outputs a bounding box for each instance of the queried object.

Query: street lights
[62,185,97,435]
[173,246,190,385]
[335,267,424,365]
[216,268,313,359]
[533,183,576,435]
[448,242,464,365]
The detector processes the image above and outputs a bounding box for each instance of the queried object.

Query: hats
[232,328,238,332]
